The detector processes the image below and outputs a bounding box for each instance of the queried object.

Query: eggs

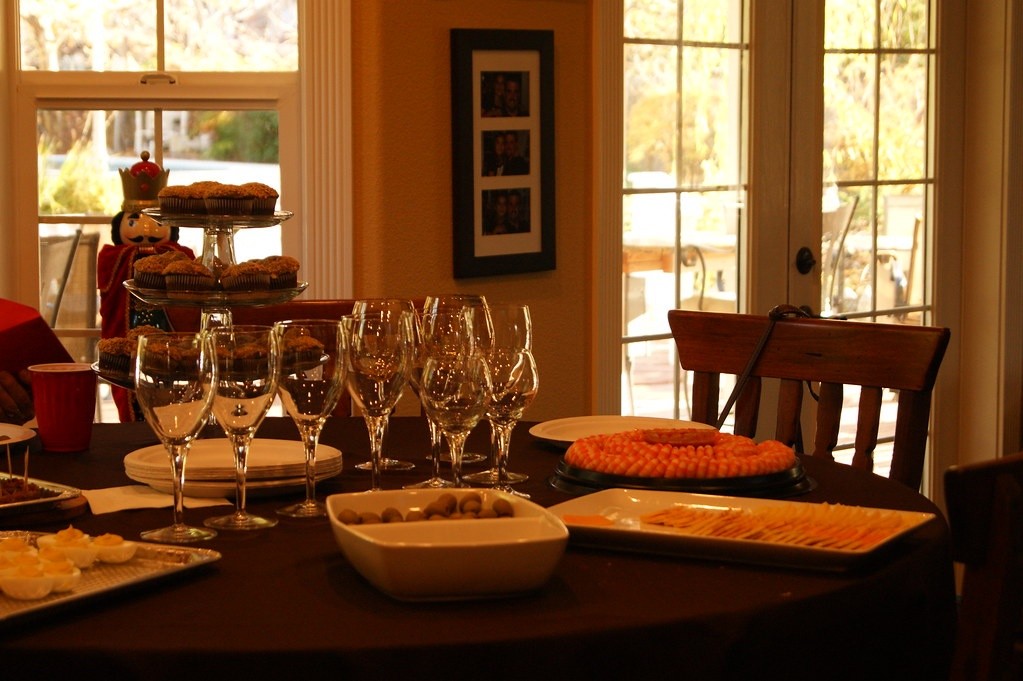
[0,524,137,600]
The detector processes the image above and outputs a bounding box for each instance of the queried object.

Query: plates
[529,415,719,442]
[545,487,937,558]
[123,438,344,497]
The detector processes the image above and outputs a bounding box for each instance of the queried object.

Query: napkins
[81,483,233,513]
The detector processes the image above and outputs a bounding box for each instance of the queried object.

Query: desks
[0,381,947,680]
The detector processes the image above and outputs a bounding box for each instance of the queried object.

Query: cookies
[560,500,906,554]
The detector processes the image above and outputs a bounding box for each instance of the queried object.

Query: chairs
[666,310,951,493]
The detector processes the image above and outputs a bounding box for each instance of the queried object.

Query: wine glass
[133,292,540,545]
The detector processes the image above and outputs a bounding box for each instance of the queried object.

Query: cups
[27,363,99,453]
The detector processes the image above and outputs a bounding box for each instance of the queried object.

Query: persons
[96,151,196,423]
[486,131,529,176]
[483,192,527,235]
[482,74,529,117]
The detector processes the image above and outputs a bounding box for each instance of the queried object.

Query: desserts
[98,323,325,381]
[133,251,299,305]
[157,180,279,217]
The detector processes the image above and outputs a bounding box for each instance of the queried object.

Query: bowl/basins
[325,488,569,599]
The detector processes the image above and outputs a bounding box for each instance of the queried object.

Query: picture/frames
[448,26,557,283]
